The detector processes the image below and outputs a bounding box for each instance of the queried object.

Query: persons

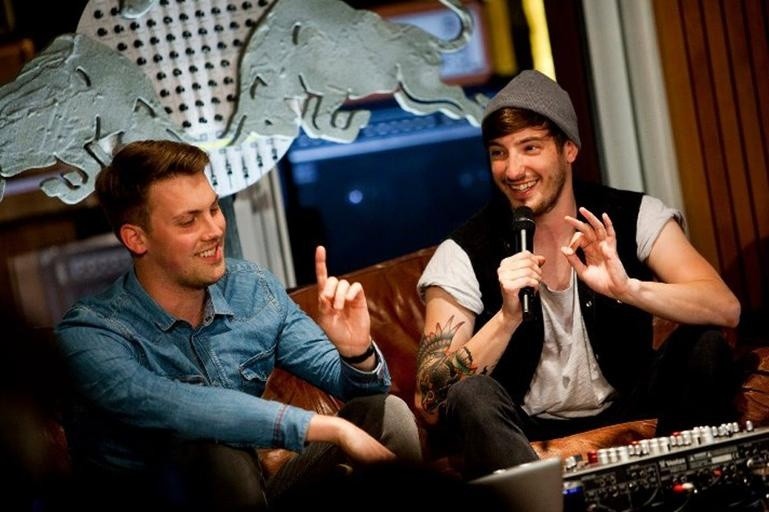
[44,137,422,511]
[413,70,742,466]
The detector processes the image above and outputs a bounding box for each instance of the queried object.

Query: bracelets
[340,343,375,365]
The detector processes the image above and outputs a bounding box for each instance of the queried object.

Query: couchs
[0,241,742,512]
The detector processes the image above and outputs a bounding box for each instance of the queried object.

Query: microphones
[511,205,537,323]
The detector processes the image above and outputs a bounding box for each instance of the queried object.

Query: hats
[481,69,582,152]
